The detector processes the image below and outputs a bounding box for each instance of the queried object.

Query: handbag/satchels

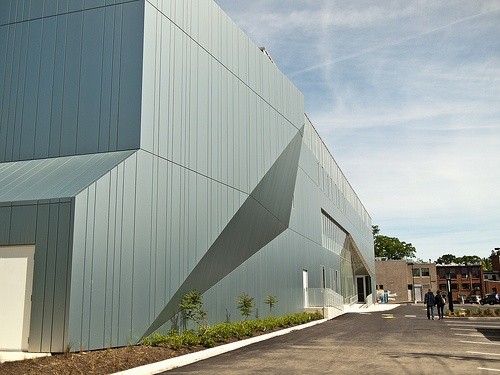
[441,295,445,304]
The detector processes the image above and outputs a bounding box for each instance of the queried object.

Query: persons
[424,289,436,320]
[433,290,445,320]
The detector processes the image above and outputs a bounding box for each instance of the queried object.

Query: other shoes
[428,317,434,320]
[438,317,443,320]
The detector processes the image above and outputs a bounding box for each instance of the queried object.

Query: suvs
[469,294,482,304]
[480,294,499,305]
